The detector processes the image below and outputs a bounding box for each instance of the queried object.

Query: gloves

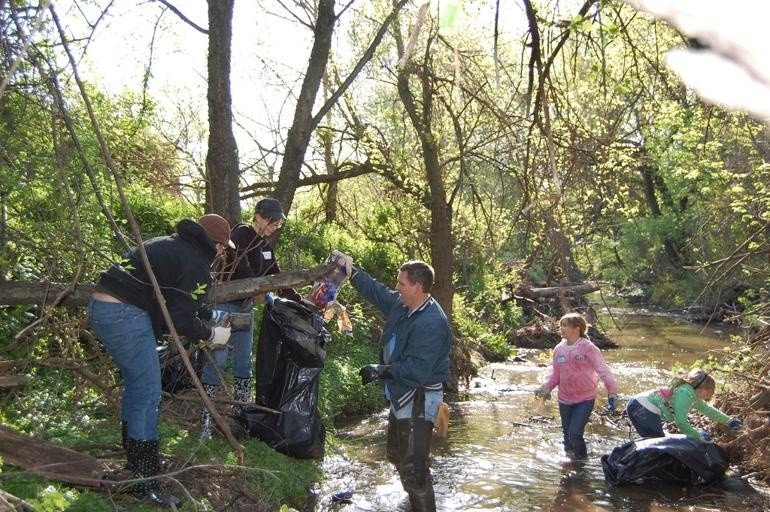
[357,364,383,386]
[207,327,232,346]
[210,309,232,328]
[606,392,617,412]
[700,431,713,443]
[725,415,743,431]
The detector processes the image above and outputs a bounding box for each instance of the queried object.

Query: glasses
[262,216,282,229]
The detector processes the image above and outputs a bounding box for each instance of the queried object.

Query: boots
[122,417,172,473]
[233,376,253,415]
[125,434,183,509]
[199,382,220,440]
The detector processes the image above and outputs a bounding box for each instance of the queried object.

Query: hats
[197,213,236,250]
[255,198,287,220]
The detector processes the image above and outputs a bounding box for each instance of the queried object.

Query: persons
[86,213,236,507]
[533,313,620,459]
[336,256,454,512]
[198,197,315,450]
[627,368,741,444]
